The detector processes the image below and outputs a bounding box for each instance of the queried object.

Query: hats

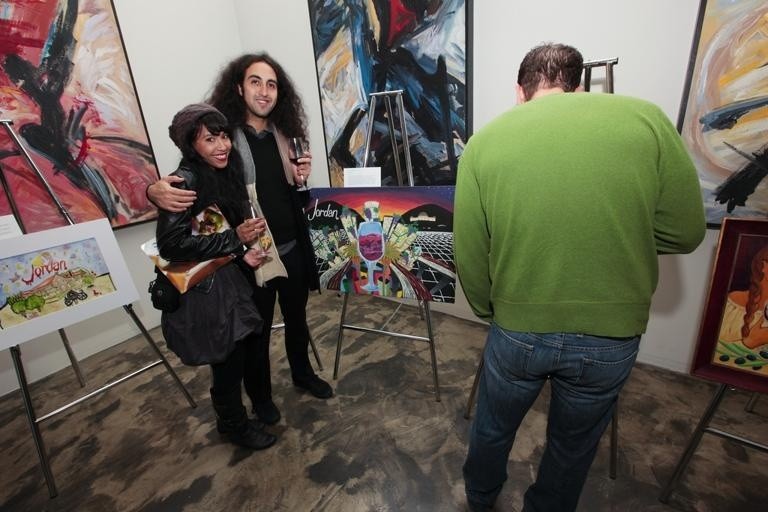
[168,102,228,146]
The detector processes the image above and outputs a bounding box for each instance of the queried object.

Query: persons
[446,40,708,507]
[140,48,335,427]
[154,103,279,453]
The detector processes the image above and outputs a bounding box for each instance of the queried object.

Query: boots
[212,400,276,449]
[244,383,280,424]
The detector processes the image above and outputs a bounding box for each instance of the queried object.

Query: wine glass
[357,220,386,292]
[287,135,313,192]
[242,199,273,260]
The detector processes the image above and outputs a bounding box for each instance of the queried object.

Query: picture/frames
[307,0,475,191]
[1,0,166,231]
[688,216,768,395]
[671,0,768,231]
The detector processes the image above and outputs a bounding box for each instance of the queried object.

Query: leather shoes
[294,375,334,400]
